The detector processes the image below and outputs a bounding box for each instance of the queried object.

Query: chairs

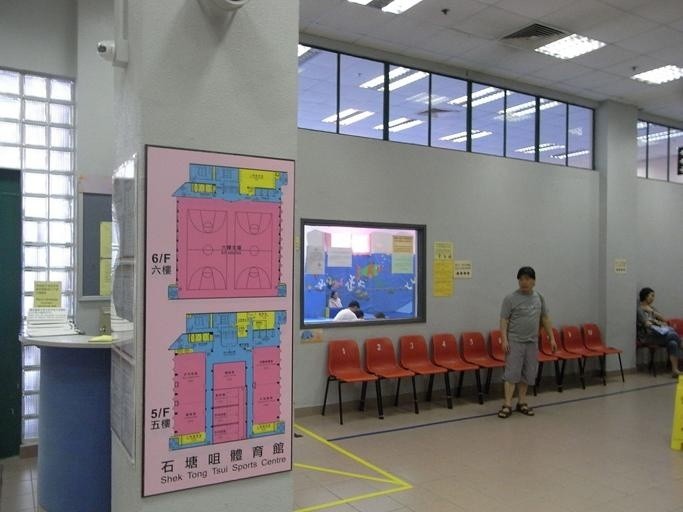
[430,334,483,408]
[489,330,536,396]
[321,339,382,426]
[582,323,625,384]
[539,327,585,391]
[460,331,507,399]
[364,336,419,417]
[560,326,606,389]
[397,335,453,414]
[634,316,682,379]
[536,351,561,397]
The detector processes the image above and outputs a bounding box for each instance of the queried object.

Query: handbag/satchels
[650,324,675,335]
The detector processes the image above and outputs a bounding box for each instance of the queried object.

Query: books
[27,309,75,336]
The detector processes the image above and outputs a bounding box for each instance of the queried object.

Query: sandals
[498,405,512,418]
[516,402,535,416]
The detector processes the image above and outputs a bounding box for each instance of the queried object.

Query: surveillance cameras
[96,40,115,60]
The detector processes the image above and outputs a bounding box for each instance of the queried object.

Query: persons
[495,266,557,419]
[636,286,682,378]
[328,291,386,320]
